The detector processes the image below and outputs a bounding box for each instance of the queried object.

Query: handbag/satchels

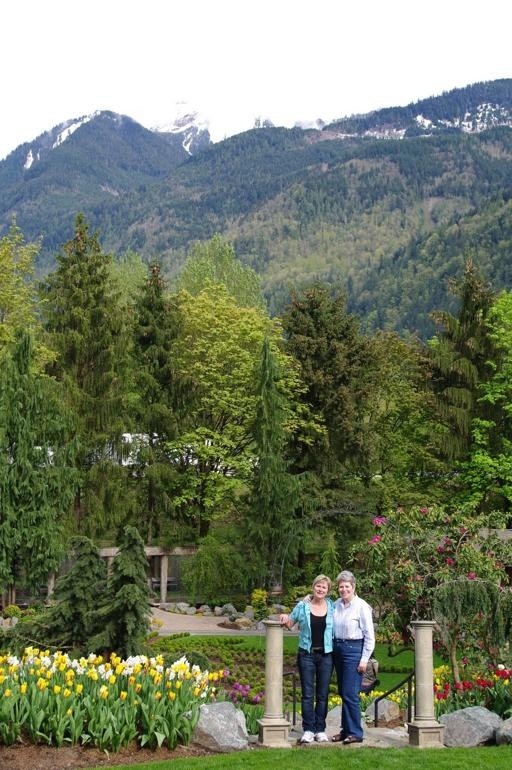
[374,679,381,687]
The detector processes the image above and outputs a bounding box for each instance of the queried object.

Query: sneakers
[299,730,315,743]
[314,731,330,743]
[331,731,347,742]
[343,734,364,744]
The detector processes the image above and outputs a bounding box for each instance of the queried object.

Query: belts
[310,646,325,654]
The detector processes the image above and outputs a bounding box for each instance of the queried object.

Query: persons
[280,573,337,743]
[360,654,378,696]
[332,571,376,745]
